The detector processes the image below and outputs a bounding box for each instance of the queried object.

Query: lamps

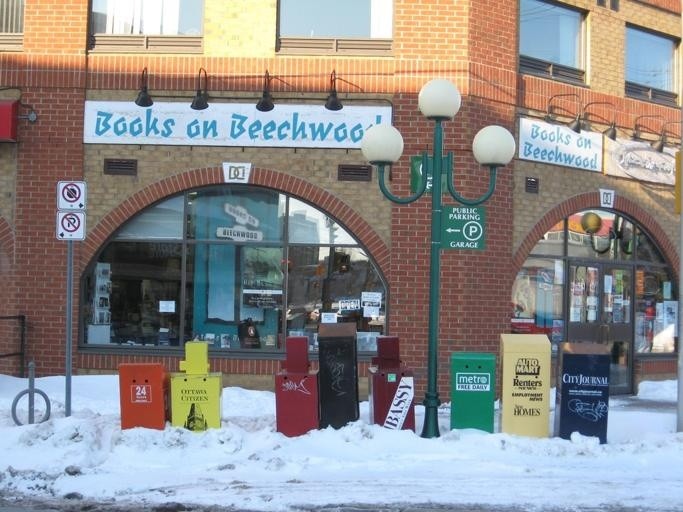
[135,68,364,112]
[545,92,667,152]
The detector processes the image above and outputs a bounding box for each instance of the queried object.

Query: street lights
[361,77,517,439]
[582,212,648,395]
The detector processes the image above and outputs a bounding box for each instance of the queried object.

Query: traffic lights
[334,252,352,273]
